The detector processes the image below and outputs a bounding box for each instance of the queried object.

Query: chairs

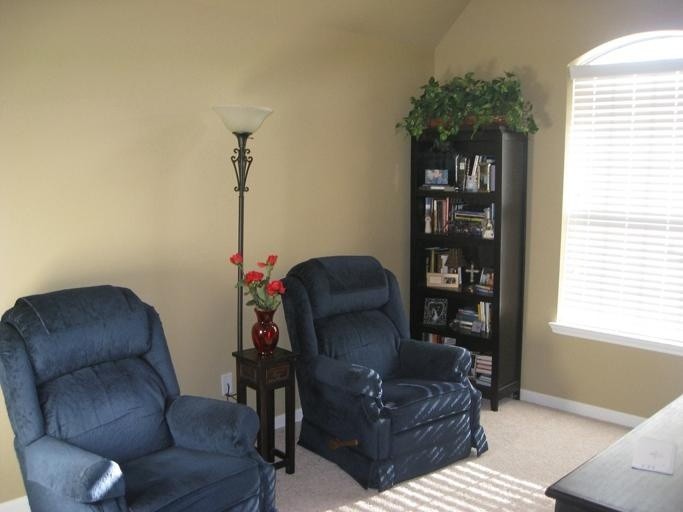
[281,254,488,492]
[0,284,274,512]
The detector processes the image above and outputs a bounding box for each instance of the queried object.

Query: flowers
[229,252,287,311]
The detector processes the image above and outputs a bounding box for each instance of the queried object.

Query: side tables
[233,347,299,476]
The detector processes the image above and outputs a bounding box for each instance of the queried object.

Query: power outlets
[221,372,233,397]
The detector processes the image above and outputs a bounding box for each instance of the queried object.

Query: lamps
[215,105,272,355]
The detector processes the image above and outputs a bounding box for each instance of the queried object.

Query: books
[417,150,494,387]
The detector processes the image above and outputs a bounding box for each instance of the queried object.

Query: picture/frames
[422,297,447,327]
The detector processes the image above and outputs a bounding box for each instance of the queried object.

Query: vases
[249,307,281,358]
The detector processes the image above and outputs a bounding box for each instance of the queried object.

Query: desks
[543,395,683,512]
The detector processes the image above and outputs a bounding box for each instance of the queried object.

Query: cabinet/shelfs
[409,129,527,411]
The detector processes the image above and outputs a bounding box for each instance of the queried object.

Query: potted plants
[393,68,539,138]
